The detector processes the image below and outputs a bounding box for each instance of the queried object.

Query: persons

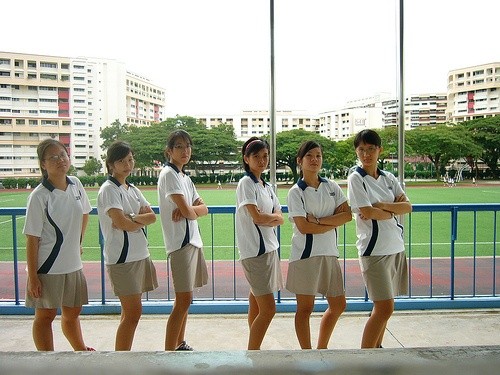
[155,129,209,352]
[285,141,354,350]
[20,137,97,352]
[345,128,413,349]
[96,140,160,352]
[443,176,478,188]
[234,136,283,350]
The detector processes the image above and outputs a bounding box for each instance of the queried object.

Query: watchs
[314,217,321,226]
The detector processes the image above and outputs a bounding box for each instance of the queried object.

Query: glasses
[44,152,68,161]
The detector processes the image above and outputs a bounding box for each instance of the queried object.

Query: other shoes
[175,341,195,351]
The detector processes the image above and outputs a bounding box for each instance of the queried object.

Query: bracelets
[129,211,137,222]
